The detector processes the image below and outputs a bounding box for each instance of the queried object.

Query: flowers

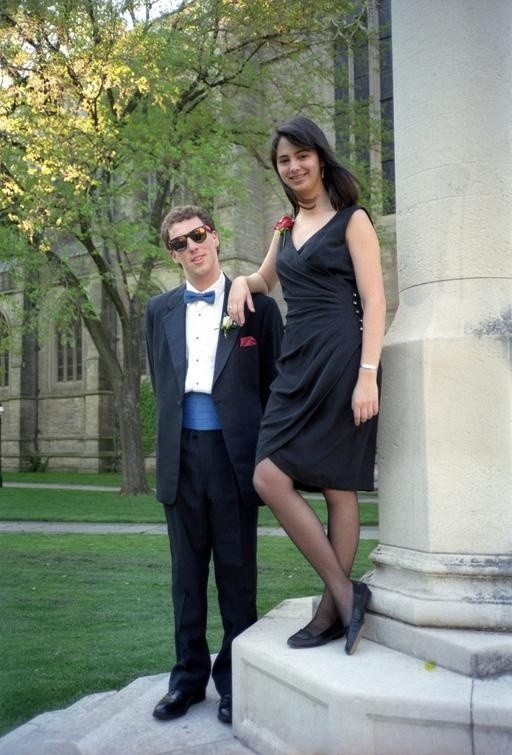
[274,214,294,248]
[213,313,238,339]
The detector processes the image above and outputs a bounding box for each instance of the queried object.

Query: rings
[228,306,231,308]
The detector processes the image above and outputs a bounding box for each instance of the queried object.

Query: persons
[226,117,386,654]
[144,207,284,723]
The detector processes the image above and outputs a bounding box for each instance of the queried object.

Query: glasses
[167,225,212,252]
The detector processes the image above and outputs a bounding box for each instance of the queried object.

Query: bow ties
[183,290,215,304]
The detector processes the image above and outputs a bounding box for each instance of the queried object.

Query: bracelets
[359,364,378,371]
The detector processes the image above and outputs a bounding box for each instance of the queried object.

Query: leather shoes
[153,689,205,720]
[344,579,371,655]
[218,695,232,722]
[287,616,344,647]
